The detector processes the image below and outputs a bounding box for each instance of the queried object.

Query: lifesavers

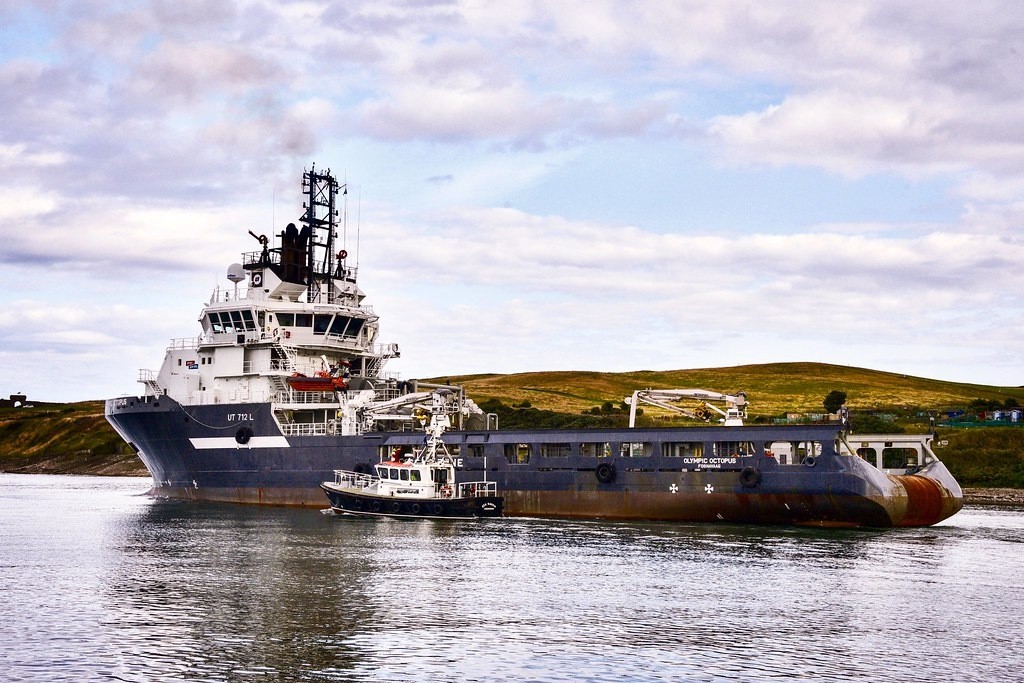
[738,466,760,488]
[273,328,278,337]
[373,502,380,510]
[392,503,400,512]
[327,421,337,434]
[595,462,615,483]
[339,250,347,258]
[355,500,362,510]
[441,485,452,497]
[393,344,398,352]
[412,504,420,513]
[235,427,249,444]
[433,505,443,514]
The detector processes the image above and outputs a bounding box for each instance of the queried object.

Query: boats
[103,157,968,531]
[316,428,506,521]
[285,370,346,392]
